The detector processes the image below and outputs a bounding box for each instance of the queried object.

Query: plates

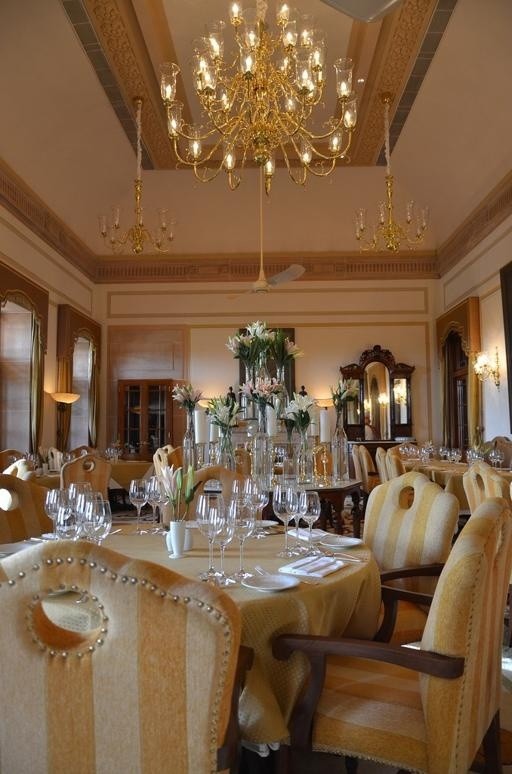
[42,532,59,540]
[320,537,362,550]
[241,575,300,593]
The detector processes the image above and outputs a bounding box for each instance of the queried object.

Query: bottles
[154,438,160,451]
[166,528,192,551]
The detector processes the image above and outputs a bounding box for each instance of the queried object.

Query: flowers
[172,320,360,477]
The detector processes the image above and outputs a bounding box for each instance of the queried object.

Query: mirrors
[339,343,416,441]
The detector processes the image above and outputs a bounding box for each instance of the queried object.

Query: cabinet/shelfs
[117,379,190,460]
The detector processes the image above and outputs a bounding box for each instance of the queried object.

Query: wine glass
[194,478,321,589]
[399,446,434,462]
[105,448,119,463]
[44,481,112,545]
[129,477,170,535]
[438,446,505,472]
[274,446,284,466]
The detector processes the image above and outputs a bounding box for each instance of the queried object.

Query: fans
[228,161,306,310]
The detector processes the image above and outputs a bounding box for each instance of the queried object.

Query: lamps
[160,0,358,196]
[473,347,500,387]
[98,95,178,255]
[50,392,81,454]
[352,92,432,254]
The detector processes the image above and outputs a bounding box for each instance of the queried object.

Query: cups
[394,437,415,441]
[7,454,17,464]
[22,453,48,478]
[60,452,72,464]
